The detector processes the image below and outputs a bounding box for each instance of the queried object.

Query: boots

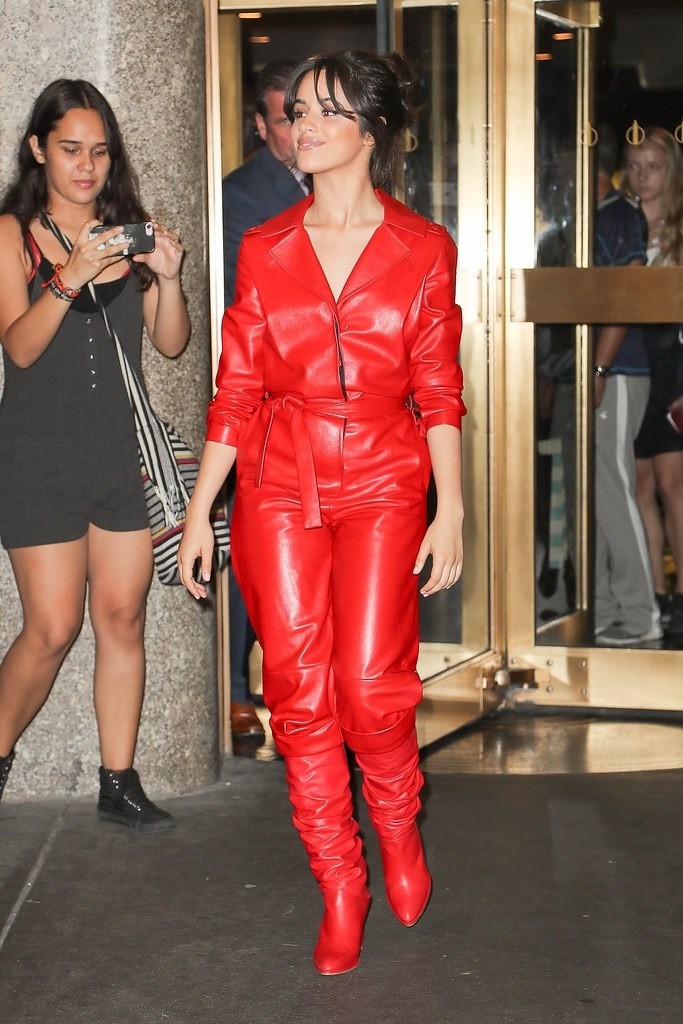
[341,706,433,926]
[274,723,372,976]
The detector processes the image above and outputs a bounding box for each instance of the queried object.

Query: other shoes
[656,593,671,623]
[96,761,177,832]
[597,621,664,645]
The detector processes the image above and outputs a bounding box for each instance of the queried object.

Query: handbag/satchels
[134,412,233,588]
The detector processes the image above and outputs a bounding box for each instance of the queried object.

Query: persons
[222,59,682,749]
[0,78,191,835]
[179,51,467,972]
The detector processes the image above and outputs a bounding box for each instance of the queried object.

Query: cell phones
[90,222,155,258]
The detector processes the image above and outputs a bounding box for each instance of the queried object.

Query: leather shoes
[231,699,266,755]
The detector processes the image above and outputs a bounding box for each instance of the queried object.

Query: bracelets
[46,265,80,304]
[593,365,612,379]
